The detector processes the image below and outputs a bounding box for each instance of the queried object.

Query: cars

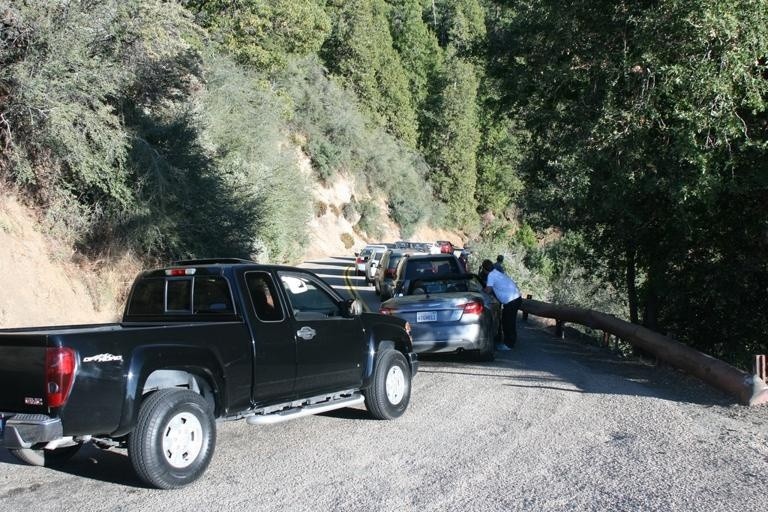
[354,240,471,292]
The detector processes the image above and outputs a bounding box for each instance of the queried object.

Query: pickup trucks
[0,254,414,490]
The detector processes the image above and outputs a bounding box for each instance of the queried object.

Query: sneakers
[496,344,512,351]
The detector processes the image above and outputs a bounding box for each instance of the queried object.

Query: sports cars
[379,272,504,362]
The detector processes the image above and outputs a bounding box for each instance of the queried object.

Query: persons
[262,281,275,307]
[480,258,521,352]
[493,254,505,274]
